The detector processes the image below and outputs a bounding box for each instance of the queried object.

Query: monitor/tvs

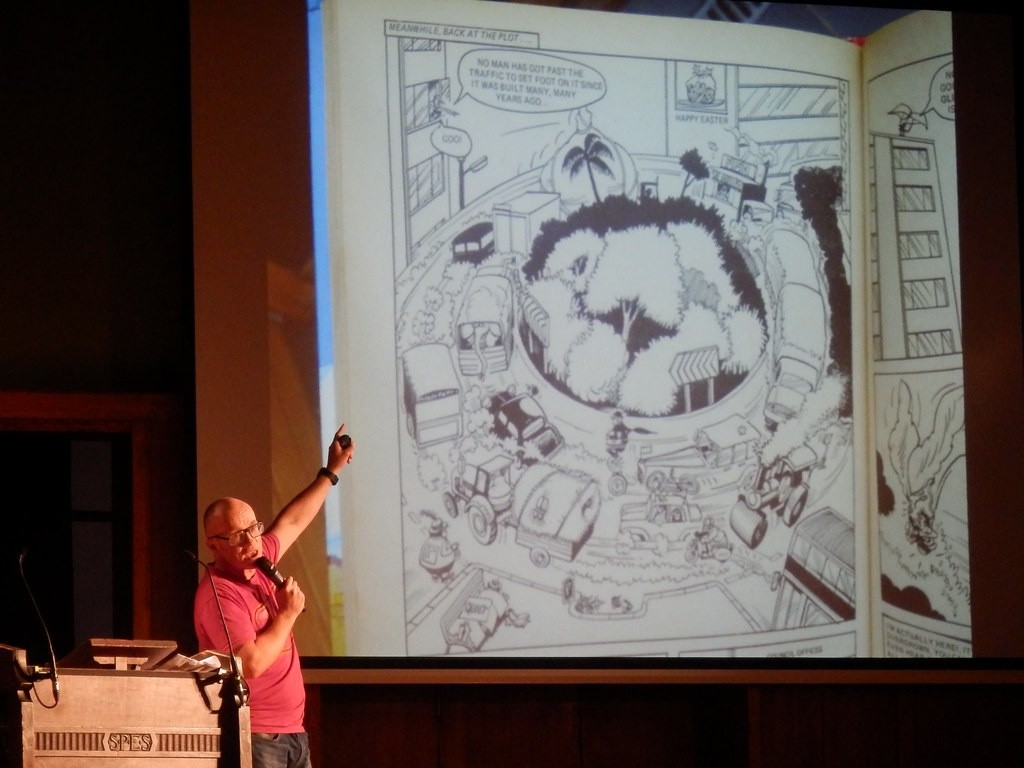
[56,638,177,669]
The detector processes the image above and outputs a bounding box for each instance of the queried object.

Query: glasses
[209,521,264,547]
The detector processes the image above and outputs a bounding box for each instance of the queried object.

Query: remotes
[339,435,351,450]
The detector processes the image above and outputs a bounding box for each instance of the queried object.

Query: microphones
[254,556,306,613]
[17,548,58,702]
[184,548,240,682]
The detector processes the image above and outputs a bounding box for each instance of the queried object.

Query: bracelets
[317,468,339,485]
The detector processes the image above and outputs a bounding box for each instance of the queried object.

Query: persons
[195,422,355,768]
